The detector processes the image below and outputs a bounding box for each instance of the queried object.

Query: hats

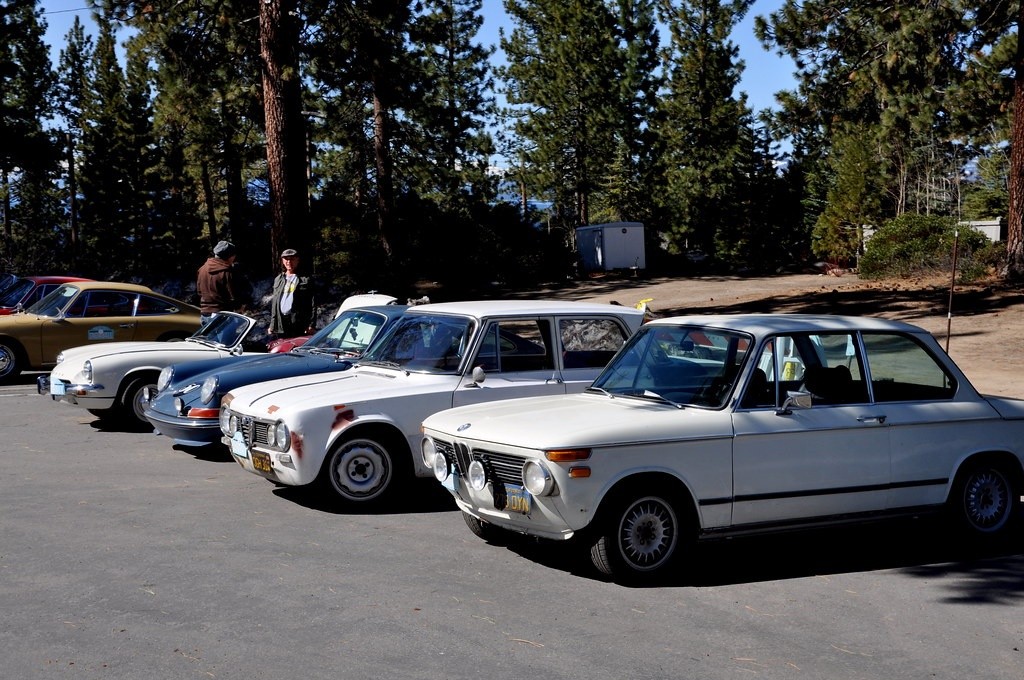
[279,247,298,258]
[212,240,238,259]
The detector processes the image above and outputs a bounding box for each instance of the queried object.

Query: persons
[197,241,244,328]
[268,249,318,338]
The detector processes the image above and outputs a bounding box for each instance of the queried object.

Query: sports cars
[37,311,282,434]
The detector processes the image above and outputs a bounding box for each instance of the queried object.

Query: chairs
[722,363,852,409]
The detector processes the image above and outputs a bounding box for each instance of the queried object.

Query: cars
[0,276,117,316]
[0,274,21,296]
[263,335,312,356]
[0,282,214,386]
[143,306,544,453]
[220,300,745,513]
[421,313,1024,586]
